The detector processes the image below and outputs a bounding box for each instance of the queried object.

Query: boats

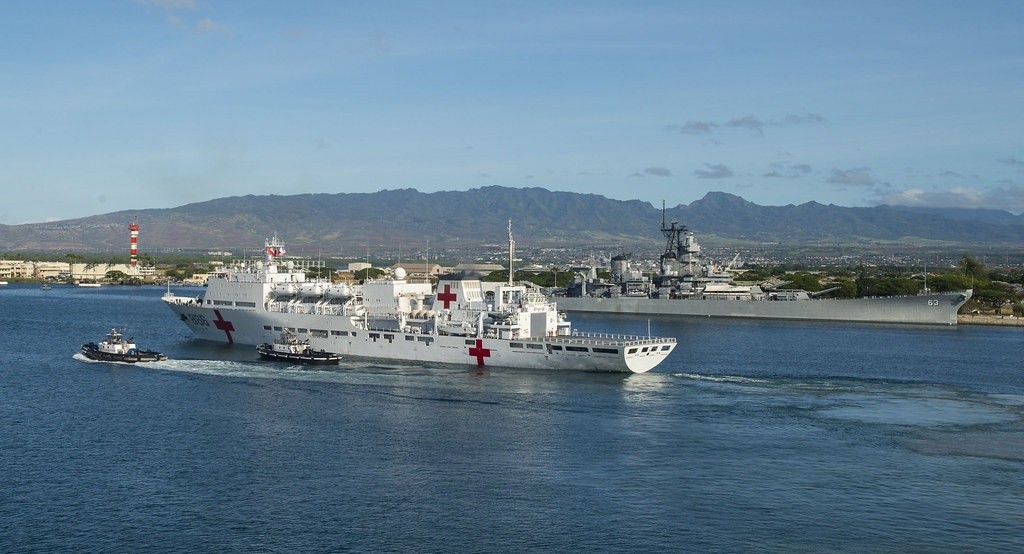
[256,326,342,364]
[79,277,101,287]
[81,327,166,363]
[43,283,51,290]
[98,273,130,285]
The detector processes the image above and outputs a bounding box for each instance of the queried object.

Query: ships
[539,197,973,325]
[162,231,678,373]
